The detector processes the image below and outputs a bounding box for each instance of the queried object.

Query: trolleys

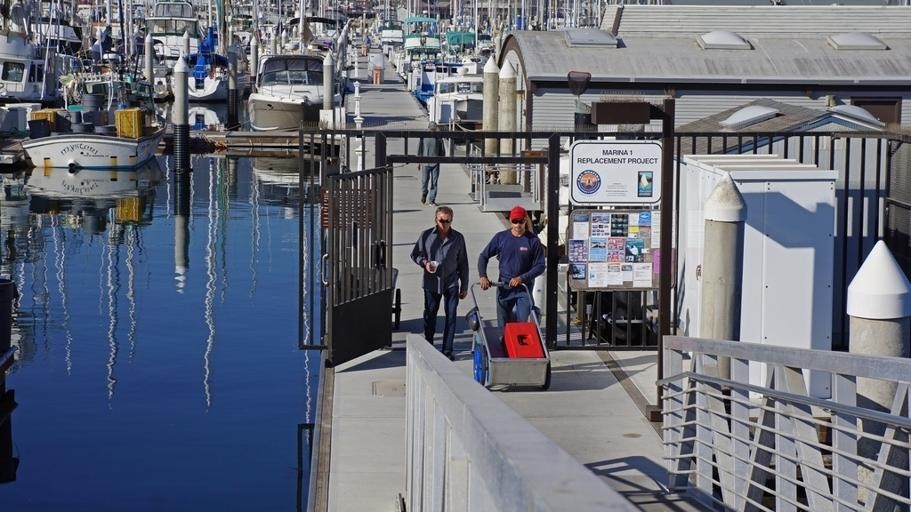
[467,281,552,391]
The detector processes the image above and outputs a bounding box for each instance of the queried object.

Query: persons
[410,207,469,361]
[418,121,445,207]
[478,206,546,326]
[363,35,370,45]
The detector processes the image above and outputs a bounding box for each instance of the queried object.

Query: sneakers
[444,352,455,361]
[422,191,438,207]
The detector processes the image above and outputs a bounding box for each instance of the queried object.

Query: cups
[431,261,439,273]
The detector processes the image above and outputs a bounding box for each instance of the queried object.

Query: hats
[510,207,527,221]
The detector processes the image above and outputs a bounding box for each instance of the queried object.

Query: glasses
[439,218,451,224]
[512,220,525,224]
